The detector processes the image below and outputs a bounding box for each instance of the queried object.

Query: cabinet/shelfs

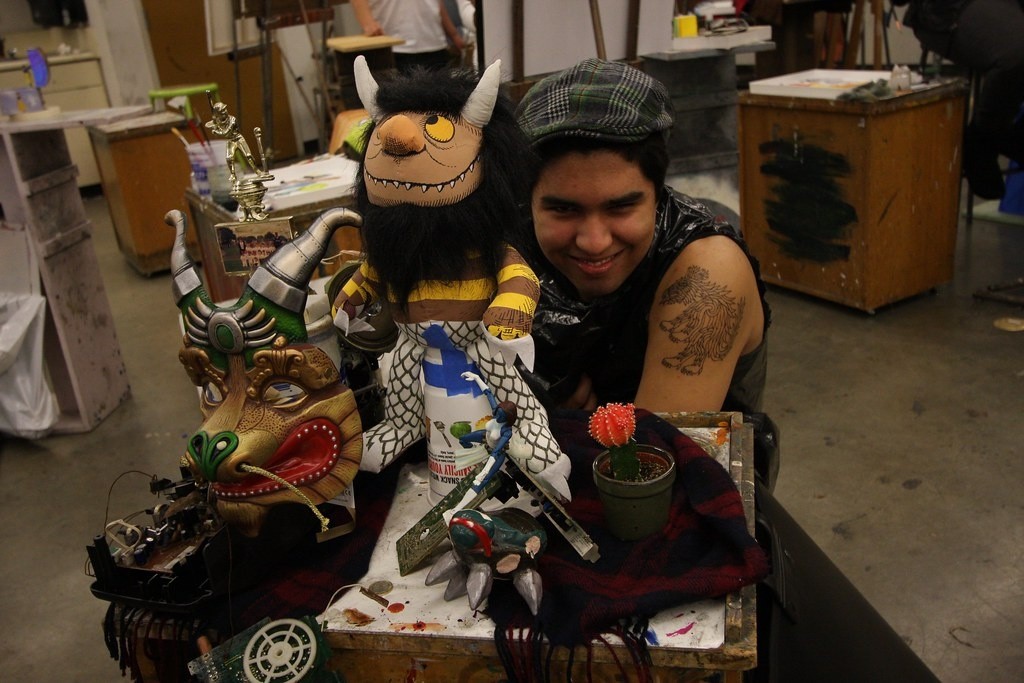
[84,108,201,275]
[321,408,758,683]
[643,40,777,173]
[0,52,113,189]
[0,105,155,433]
[185,187,361,302]
[738,79,964,310]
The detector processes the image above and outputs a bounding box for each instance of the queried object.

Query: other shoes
[962,129,1007,199]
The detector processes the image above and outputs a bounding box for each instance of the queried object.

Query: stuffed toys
[332,54,575,501]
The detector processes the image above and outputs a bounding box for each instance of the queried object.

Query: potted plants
[587,404,676,537]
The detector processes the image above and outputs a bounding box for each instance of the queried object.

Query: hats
[515,58,673,153]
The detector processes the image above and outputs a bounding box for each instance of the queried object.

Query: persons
[205,102,264,183]
[352,0,472,83]
[513,57,782,512]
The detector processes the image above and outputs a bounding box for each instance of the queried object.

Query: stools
[149,84,249,174]
